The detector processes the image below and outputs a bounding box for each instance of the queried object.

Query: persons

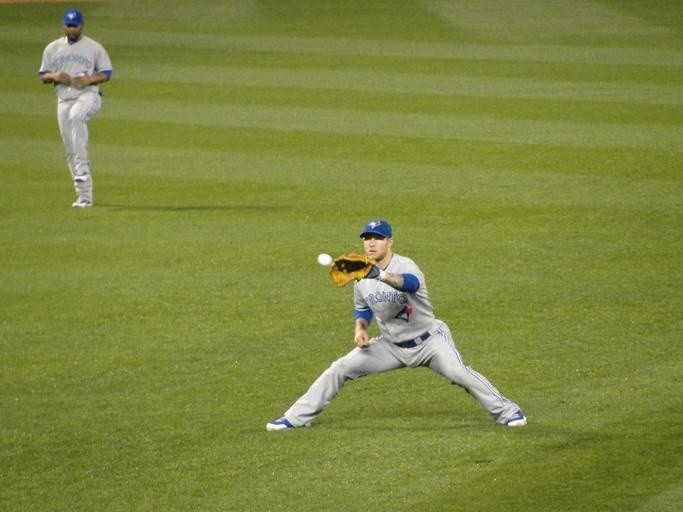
[265,220,528,429]
[39,9,112,208]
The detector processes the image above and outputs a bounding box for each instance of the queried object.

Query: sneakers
[266,417,294,431]
[72,201,92,207]
[74,173,89,181]
[507,411,527,426]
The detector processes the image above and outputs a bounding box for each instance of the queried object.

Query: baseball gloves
[329,253,378,288]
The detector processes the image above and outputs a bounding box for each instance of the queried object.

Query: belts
[394,331,430,347]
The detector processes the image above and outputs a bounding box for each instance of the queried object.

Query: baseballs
[317,254,331,267]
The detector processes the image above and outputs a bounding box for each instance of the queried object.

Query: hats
[360,220,392,238]
[64,10,82,25]
